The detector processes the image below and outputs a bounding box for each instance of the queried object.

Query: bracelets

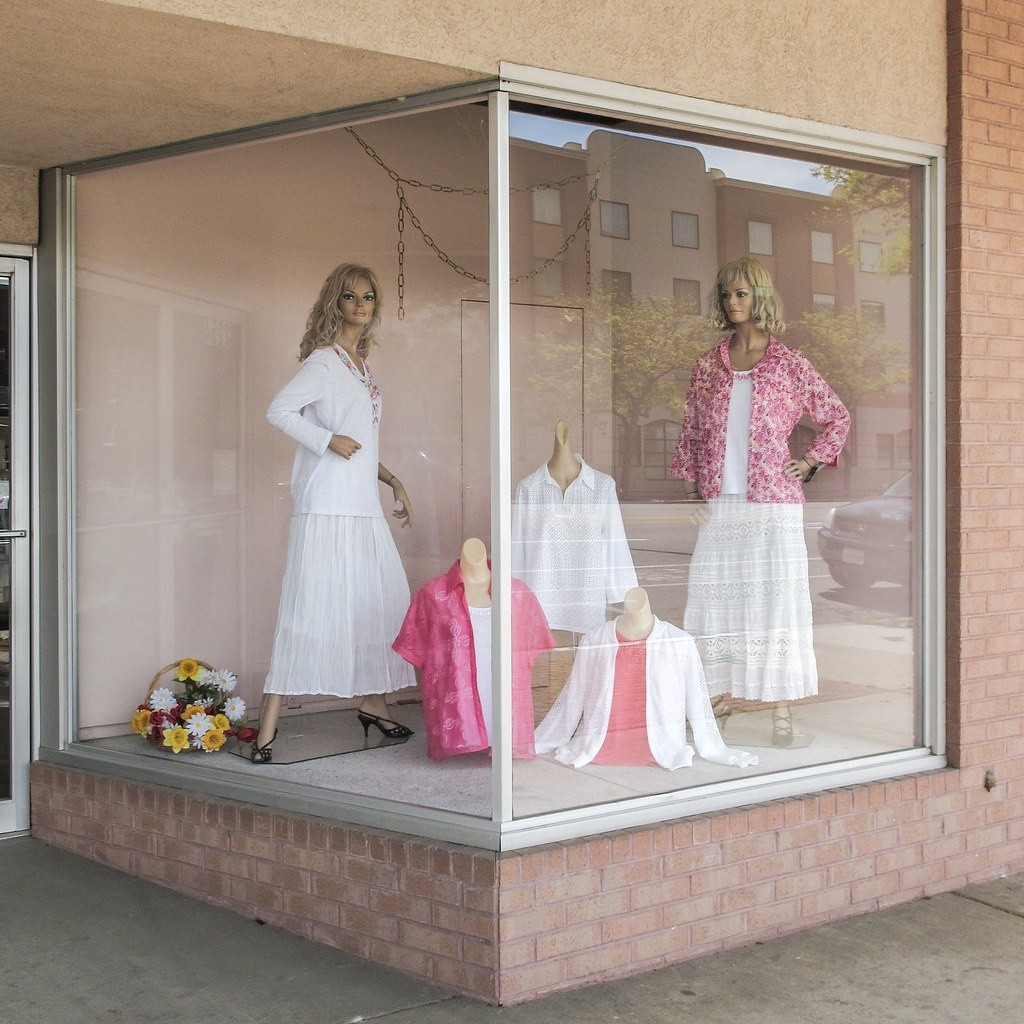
[684,490,698,497]
[388,475,395,485]
[802,457,812,468]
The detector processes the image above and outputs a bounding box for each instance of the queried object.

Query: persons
[671,257,851,743]
[391,419,760,771]
[249,264,419,766]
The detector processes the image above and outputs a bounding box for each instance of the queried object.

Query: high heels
[251,727,279,762]
[357,709,415,739]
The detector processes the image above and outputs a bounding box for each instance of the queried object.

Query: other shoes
[770,706,794,748]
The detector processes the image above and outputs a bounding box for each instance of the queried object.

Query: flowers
[131,655,246,757]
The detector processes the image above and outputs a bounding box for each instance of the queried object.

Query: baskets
[144,659,216,752]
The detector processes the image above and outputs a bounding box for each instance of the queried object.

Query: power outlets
[287,695,302,709]
